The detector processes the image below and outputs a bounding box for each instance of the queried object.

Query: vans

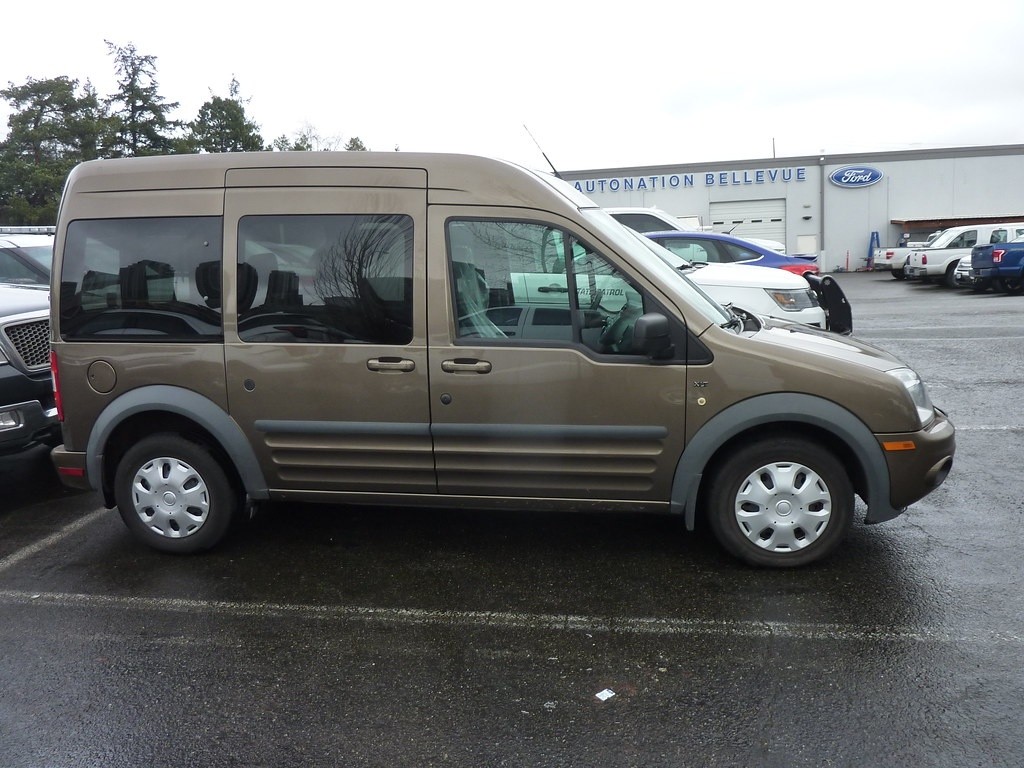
[52,148,956,568]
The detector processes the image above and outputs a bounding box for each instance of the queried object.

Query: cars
[928,223,1024,248]
[510,225,830,330]
[0,224,191,309]
[563,229,821,277]
[602,206,698,234]
[0,283,64,520]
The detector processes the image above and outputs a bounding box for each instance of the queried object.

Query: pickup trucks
[872,232,942,280]
[970,242,1024,297]
[904,246,973,289]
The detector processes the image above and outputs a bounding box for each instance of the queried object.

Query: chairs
[452,246,509,339]
